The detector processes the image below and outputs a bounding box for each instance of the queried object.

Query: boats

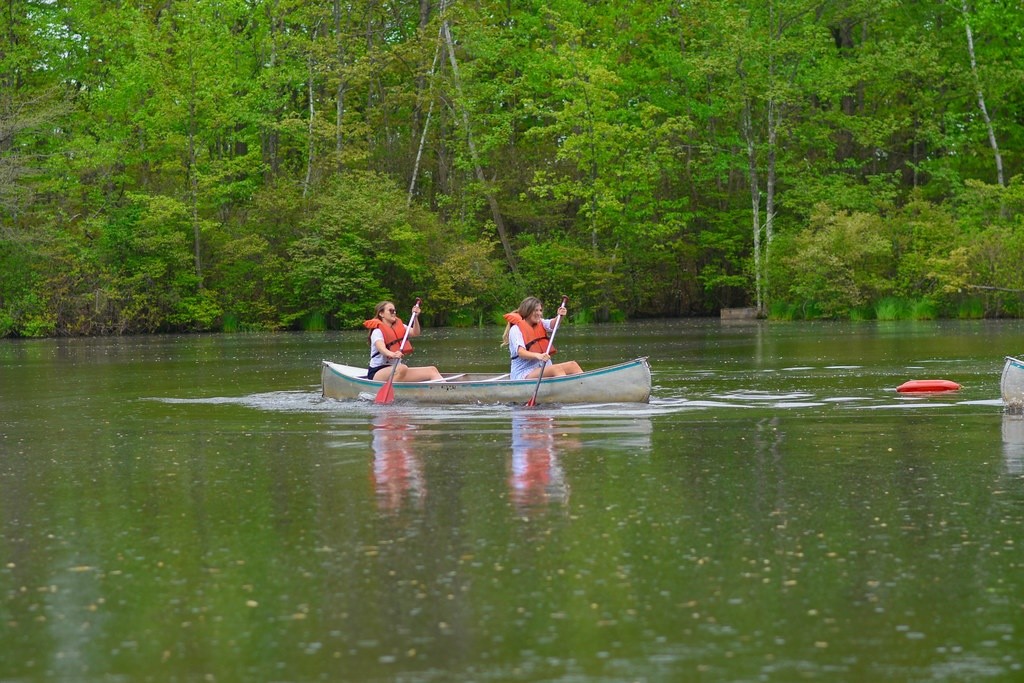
[320,355,653,406]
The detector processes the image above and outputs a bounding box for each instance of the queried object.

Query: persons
[508,297,583,378]
[368,300,448,383]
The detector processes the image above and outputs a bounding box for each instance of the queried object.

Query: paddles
[374,297,422,406]
[525,295,569,407]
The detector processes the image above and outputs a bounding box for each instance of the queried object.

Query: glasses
[383,308,397,314]
